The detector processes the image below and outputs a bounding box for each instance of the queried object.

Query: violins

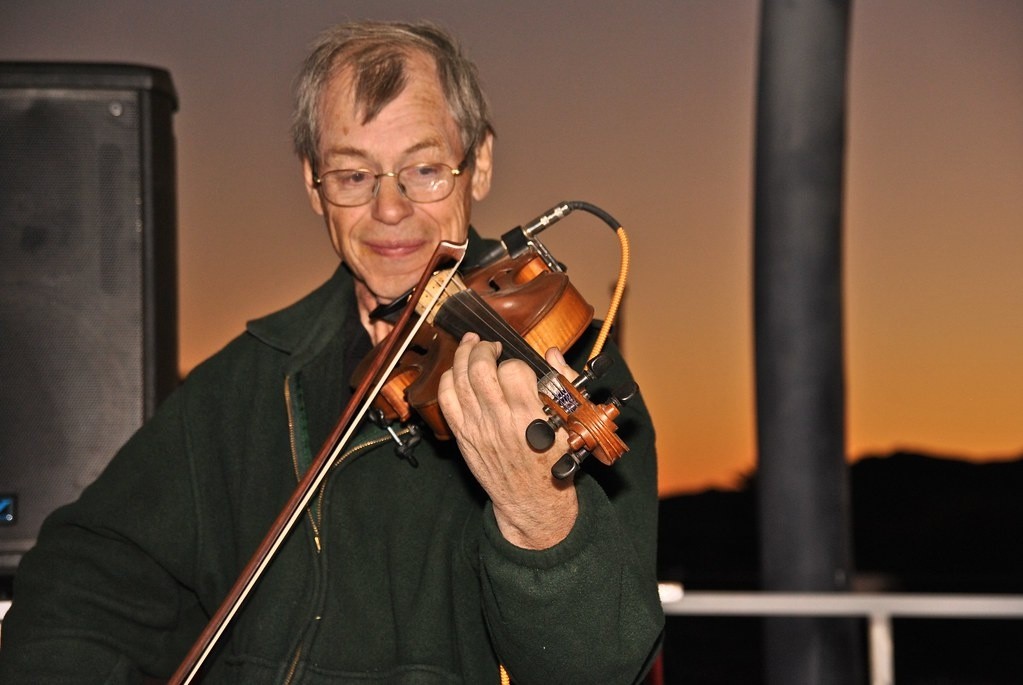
[347,241,634,486]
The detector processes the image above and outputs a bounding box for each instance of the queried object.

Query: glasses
[312,131,477,208]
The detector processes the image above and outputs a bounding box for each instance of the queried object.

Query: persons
[0,21,666,685]
